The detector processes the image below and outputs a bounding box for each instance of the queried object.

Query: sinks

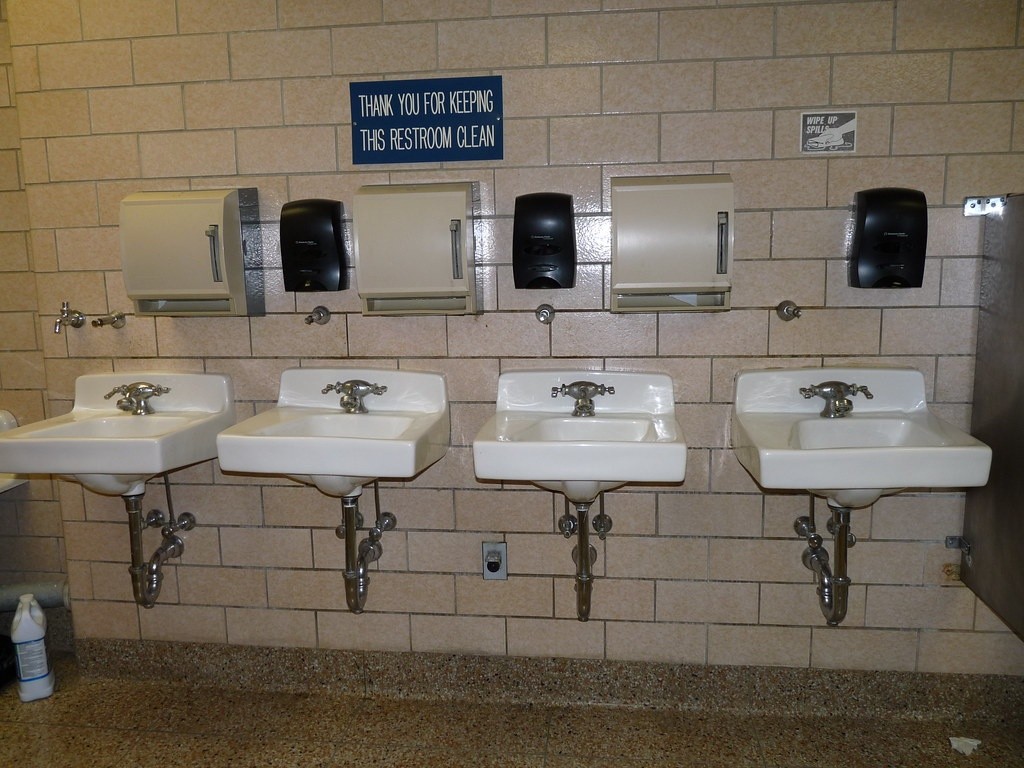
[729,364,991,509]
[1,371,238,496]
[474,369,687,503]
[215,367,450,497]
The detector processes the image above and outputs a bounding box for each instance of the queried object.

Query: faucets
[799,380,873,418]
[103,381,172,416]
[53,301,84,334]
[551,380,615,417]
[321,379,388,415]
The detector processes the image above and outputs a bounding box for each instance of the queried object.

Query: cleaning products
[10,593,56,702]
